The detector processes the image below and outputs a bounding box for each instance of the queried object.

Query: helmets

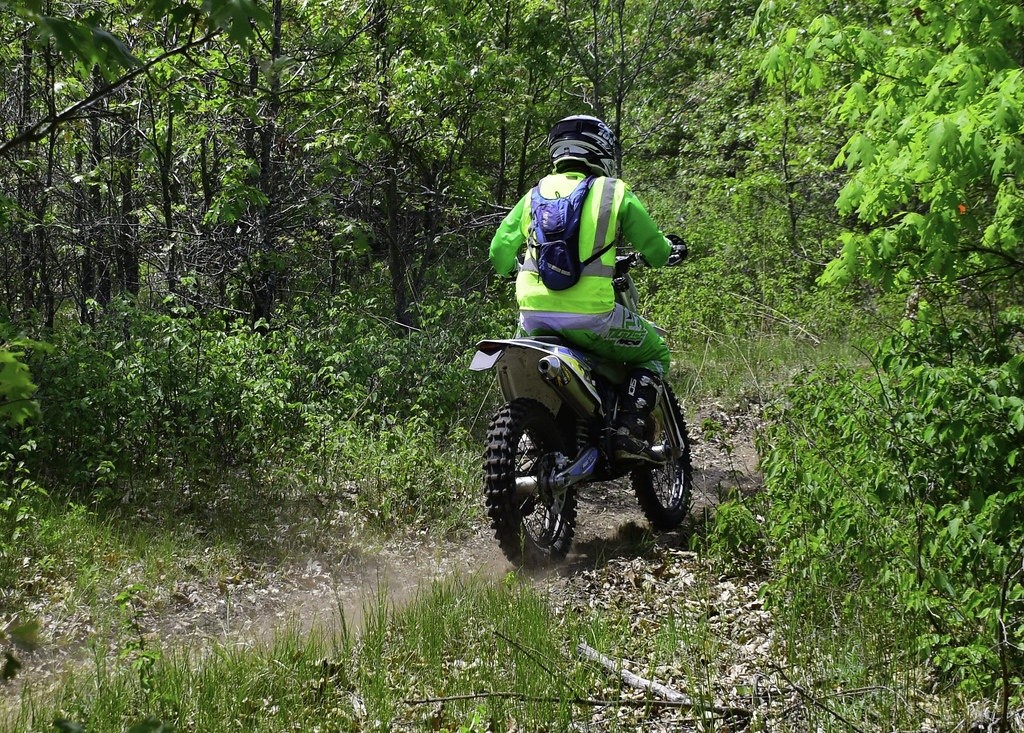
[548,113,618,178]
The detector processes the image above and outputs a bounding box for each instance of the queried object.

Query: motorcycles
[469,234,693,572]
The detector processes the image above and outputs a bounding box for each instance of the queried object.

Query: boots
[518,432,535,454]
[616,368,667,465]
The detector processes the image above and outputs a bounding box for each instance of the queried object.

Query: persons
[489,112,674,466]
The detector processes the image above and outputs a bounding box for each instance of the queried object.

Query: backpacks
[528,174,616,290]
